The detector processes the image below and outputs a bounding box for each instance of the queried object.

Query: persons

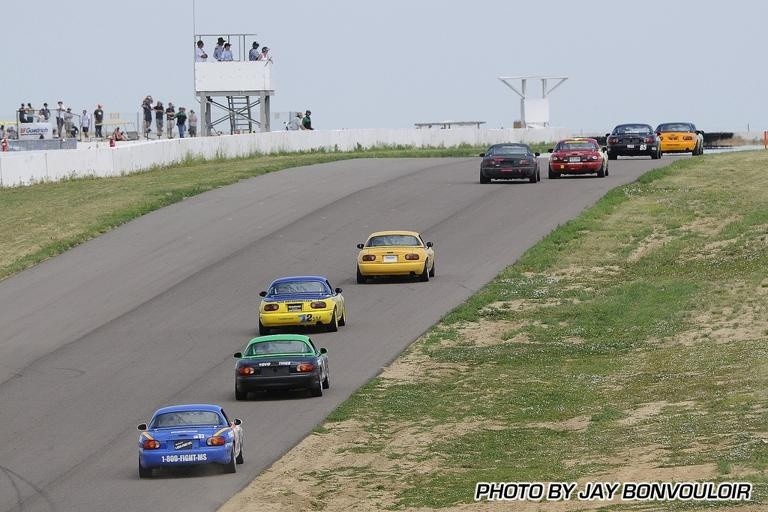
[64,108,73,137]
[80,110,90,141]
[37,108,46,122]
[142,95,153,133]
[188,109,197,137]
[285,112,306,130]
[302,110,313,130]
[153,101,164,135]
[19,103,27,122]
[196,40,208,61]
[174,107,187,138]
[165,102,175,138]
[259,47,270,60]
[213,37,225,61]
[249,41,260,60]
[43,103,51,120]
[26,102,35,123]
[112,126,128,141]
[220,43,233,61]
[0,125,5,140]
[94,104,104,138]
[56,101,65,137]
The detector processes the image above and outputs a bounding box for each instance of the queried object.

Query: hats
[305,110,311,115]
[224,43,232,48]
[252,41,260,47]
[262,47,270,52]
[297,112,303,117]
[216,37,225,44]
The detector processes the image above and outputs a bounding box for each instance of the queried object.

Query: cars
[356,231,436,282]
[606,123,661,159]
[655,122,704,157]
[234,333,330,400]
[480,143,541,183]
[548,138,608,177]
[138,402,245,478]
[258,274,346,332]
